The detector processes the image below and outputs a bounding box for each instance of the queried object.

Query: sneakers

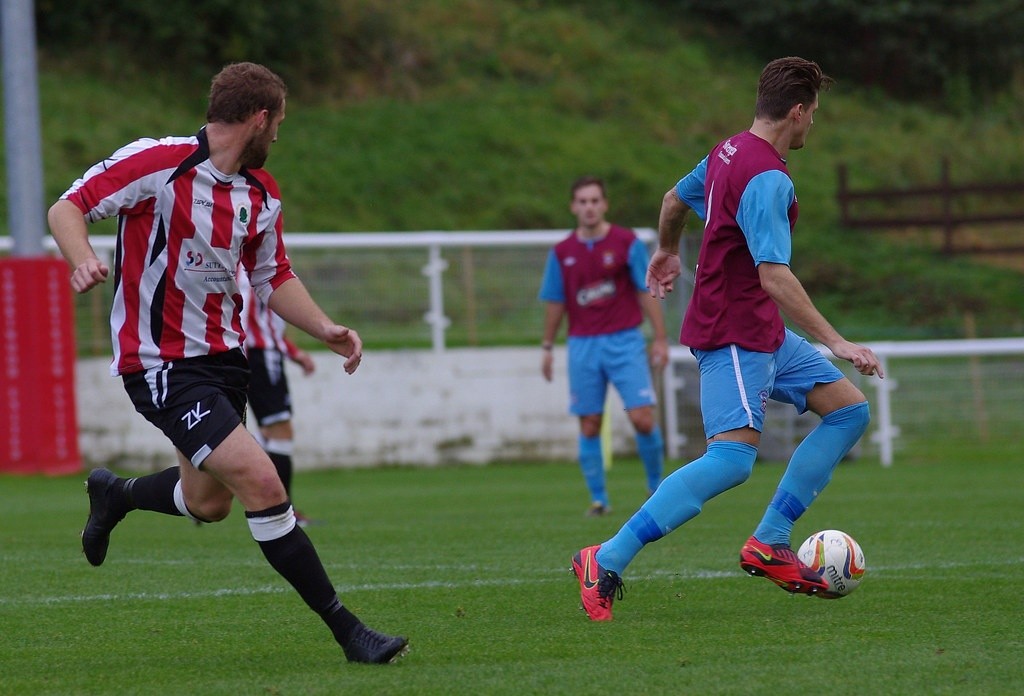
[740,535,829,596]
[585,503,613,517]
[81,466,120,567]
[572,545,615,623]
[341,622,409,664]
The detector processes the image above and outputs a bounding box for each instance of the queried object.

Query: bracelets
[542,341,552,351]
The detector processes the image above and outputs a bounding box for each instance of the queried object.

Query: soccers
[796,529,867,600]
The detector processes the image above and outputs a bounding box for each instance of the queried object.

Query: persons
[570,56,885,620]
[46,60,409,663]
[537,176,669,516]
